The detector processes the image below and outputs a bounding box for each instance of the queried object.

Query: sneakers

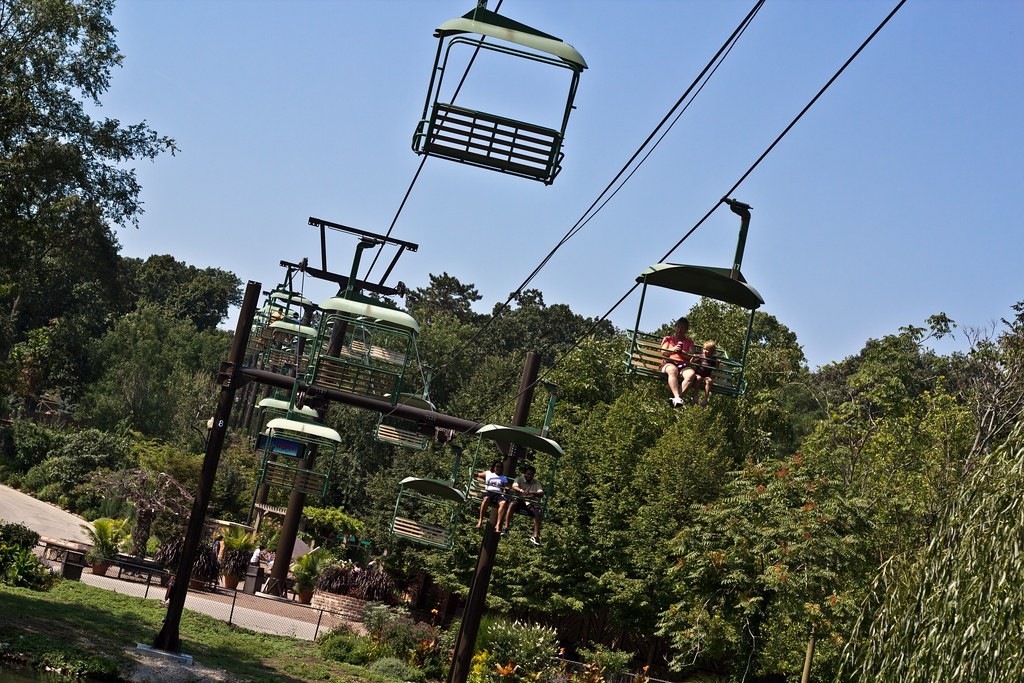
[668,397,685,408]
[530,536,541,547]
[500,528,509,538]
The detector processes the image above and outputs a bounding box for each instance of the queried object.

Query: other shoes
[493,525,501,533]
[690,394,708,407]
[476,520,483,529]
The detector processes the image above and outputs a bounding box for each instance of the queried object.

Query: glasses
[495,465,503,468]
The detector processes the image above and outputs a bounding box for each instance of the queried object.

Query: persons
[289,313,302,325]
[212,535,223,560]
[250,545,270,568]
[271,308,285,319]
[660,317,696,408]
[476,461,508,533]
[690,339,719,406]
[505,466,544,547]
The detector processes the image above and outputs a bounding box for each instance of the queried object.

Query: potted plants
[293,553,319,605]
[155,537,184,587]
[78,517,133,576]
[189,544,217,591]
[220,547,252,590]
